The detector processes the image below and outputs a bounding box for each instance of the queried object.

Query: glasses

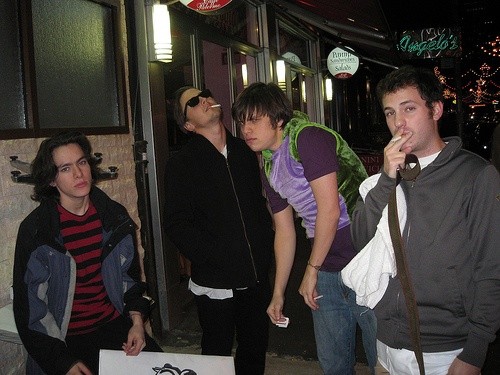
[183,88,212,122]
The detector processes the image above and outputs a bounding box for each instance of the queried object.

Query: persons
[231,82,376,375]
[159,85,278,375]
[351,66,500,375]
[13,130,167,375]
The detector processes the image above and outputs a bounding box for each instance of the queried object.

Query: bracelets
[306,260,322,271]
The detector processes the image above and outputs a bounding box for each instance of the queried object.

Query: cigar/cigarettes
[313,295,323,300]
[391,135,406,141]
[208,104,221,109]
